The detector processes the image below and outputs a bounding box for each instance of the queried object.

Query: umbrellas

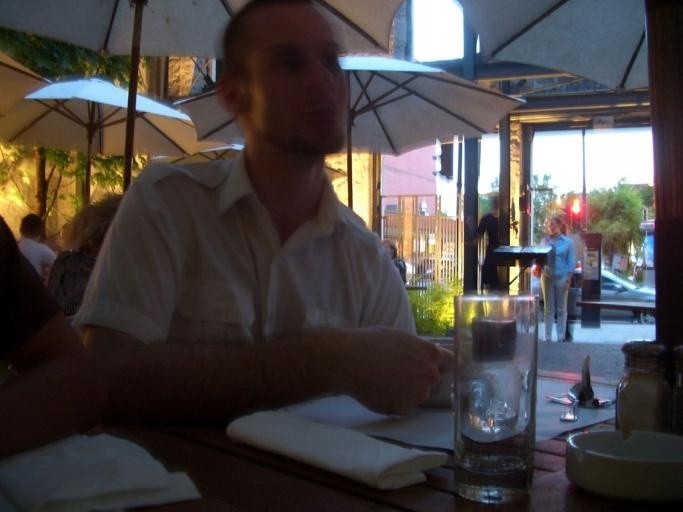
[459,0,652,91]
[0,52,235,208]
[0,0,406,194]
[172,55,527,212]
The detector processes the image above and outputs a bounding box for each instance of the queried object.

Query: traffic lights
[570,199,581,216]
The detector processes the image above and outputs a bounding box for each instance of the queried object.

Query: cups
[454,294,540,508]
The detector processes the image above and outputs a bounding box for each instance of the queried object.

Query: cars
[405,244,655,327]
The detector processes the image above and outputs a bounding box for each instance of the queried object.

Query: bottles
[613,337,683,436]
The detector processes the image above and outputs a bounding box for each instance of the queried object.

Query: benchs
[576,300,656,324]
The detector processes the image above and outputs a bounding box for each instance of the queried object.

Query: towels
[227,411,447,491]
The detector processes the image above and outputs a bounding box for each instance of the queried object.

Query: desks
[163,370,683,512]
[88,424,413,511]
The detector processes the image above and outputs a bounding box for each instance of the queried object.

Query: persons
[70,0,459,427]
[383,237,408,288]
[540,217,576,343]
[470,196,502,292]
[0,195,125,463]
